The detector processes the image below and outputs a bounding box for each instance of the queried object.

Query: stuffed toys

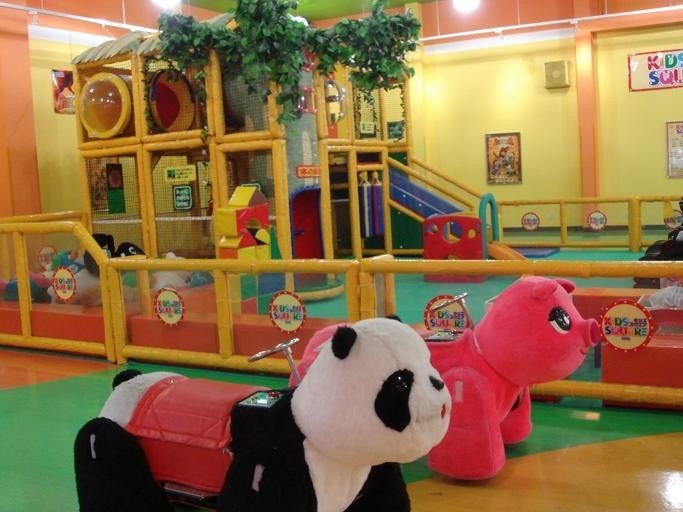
[0,233,194,306]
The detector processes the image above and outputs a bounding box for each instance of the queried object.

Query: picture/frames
[485,132,523,185]
[664,120,683,179]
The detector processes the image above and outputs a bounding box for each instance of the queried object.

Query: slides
[485,239,543,281]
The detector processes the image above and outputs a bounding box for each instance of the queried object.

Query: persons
[52,71,74,112]
